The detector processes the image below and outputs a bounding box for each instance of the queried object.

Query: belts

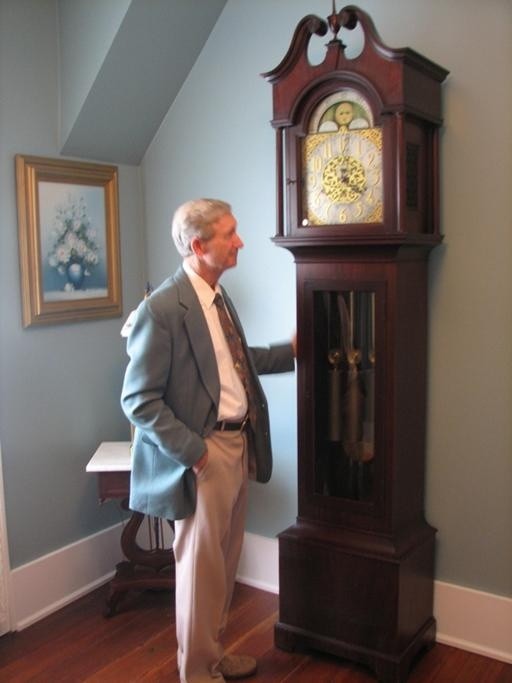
[214,415,249,433]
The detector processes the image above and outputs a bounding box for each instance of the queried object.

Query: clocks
[258,0,448,682]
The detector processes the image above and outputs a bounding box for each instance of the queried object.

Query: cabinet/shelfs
[86,440,175,618]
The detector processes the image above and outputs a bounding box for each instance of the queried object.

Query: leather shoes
[221,654,257,680]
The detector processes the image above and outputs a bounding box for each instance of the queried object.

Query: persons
[119,198,299,682]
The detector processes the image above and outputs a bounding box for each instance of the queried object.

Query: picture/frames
[14,154,124,329]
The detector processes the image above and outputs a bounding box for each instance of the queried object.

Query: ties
[215,294,258,434]
